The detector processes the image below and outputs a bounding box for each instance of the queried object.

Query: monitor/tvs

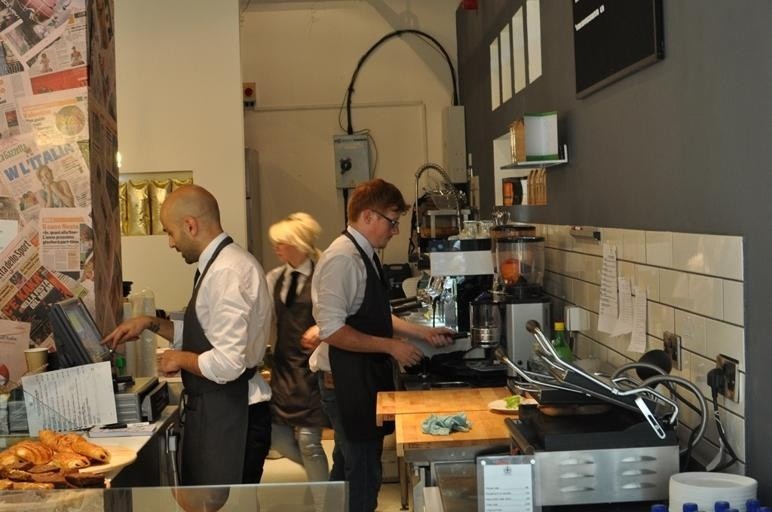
[48,296,114,368]
[572,0,665,101]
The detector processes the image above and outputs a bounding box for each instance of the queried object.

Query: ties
[285,271,300,307]
[372,253,388,289]
[193,267,201,287]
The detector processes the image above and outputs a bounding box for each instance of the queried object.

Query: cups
[24,347,48,374]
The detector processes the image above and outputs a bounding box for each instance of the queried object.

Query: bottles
[550,322,572,366]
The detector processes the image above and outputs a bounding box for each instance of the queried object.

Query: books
[21,361,156,438]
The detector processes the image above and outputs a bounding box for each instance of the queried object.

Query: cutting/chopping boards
[78,446,139,475]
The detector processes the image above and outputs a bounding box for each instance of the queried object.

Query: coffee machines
[417,208,549,374]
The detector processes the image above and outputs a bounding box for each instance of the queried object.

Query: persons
[40,54,53,73]
[70,46,85,66]
[99,185,273,486]
[80,224,94,260]
[308,179,455,512]
[264,213,334,482]
[37,165,75,209]
[170,487,230,512]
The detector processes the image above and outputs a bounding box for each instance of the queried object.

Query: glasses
[369,208,400,229]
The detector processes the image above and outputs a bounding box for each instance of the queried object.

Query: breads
[0,429,111,482]
